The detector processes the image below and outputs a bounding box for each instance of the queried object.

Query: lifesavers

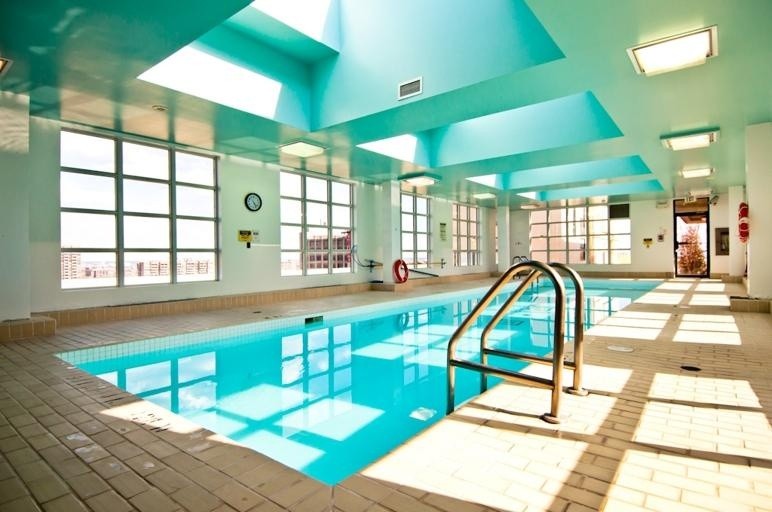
[394,259,408,282]
[738,202,749,242]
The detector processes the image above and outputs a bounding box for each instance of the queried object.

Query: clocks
[245,193,262,211]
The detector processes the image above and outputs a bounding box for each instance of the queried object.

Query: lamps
[680,168,716,179]
[625,25,719,76]
[661,130,717,151]
